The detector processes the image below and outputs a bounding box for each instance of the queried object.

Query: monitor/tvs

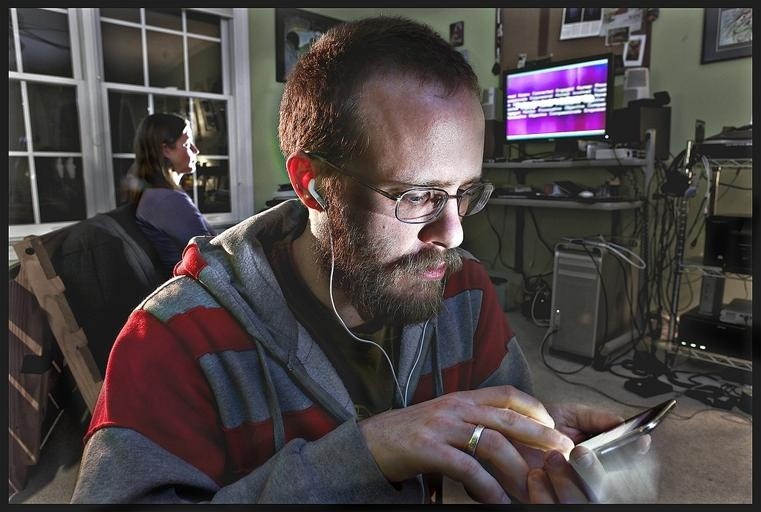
[503,52,614,153]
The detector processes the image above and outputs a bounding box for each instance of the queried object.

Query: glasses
[305,149,494,224]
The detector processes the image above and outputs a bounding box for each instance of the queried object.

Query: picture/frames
[700,7,754,65]
[449,20,464,46]
[274,7,348,82]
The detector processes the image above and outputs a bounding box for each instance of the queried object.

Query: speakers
[623,107,671,159]
[484,120,505,163]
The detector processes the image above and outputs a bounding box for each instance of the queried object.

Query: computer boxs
[549,241,639,369]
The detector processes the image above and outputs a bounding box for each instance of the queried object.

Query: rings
[464,421,486,458]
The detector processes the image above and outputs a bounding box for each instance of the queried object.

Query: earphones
[303,173,327,210]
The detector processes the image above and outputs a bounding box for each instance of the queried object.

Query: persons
[63,14,659,508]
[128,110,220,272]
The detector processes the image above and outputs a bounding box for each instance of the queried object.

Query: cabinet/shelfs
[662,124,753,380]
[481,128,656,370]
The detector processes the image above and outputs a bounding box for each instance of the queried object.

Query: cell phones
[574,399,677,456]
